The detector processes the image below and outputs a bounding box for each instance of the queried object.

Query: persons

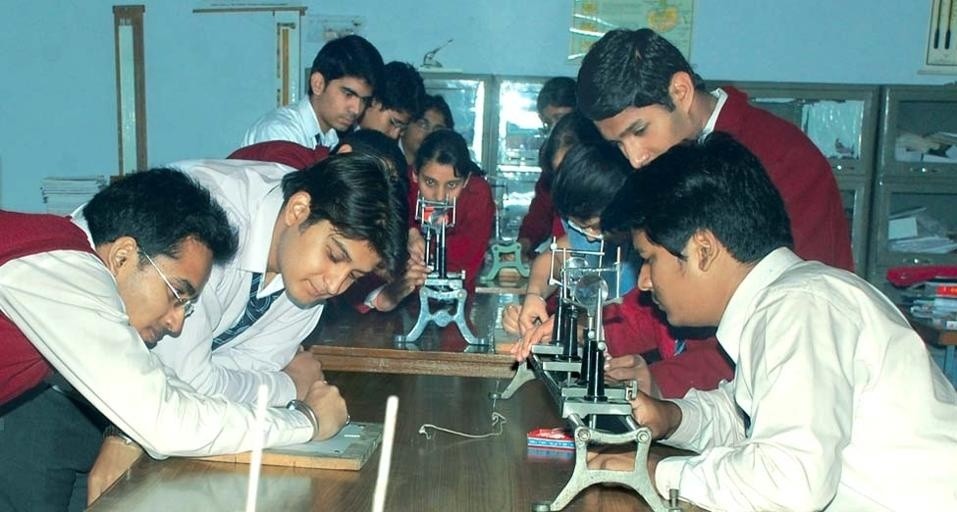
[407,126,498,306]
[397,91,456,164]
[0,148,411,512]
[0,163,352,466]
[237,32,381,153]
[511,27,854,400]
[228,126,430,315]
[572,130,957,512]
[505,76,643,337]
[359,58,427,149]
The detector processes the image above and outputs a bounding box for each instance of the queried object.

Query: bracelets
[101,422,138,455]
[289,398,320,439]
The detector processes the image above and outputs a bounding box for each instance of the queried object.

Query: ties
[212,273,285,351]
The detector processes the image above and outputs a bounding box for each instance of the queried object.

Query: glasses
[141,250,194,316]
[568,220,604,240]
[387,109,410,129]
